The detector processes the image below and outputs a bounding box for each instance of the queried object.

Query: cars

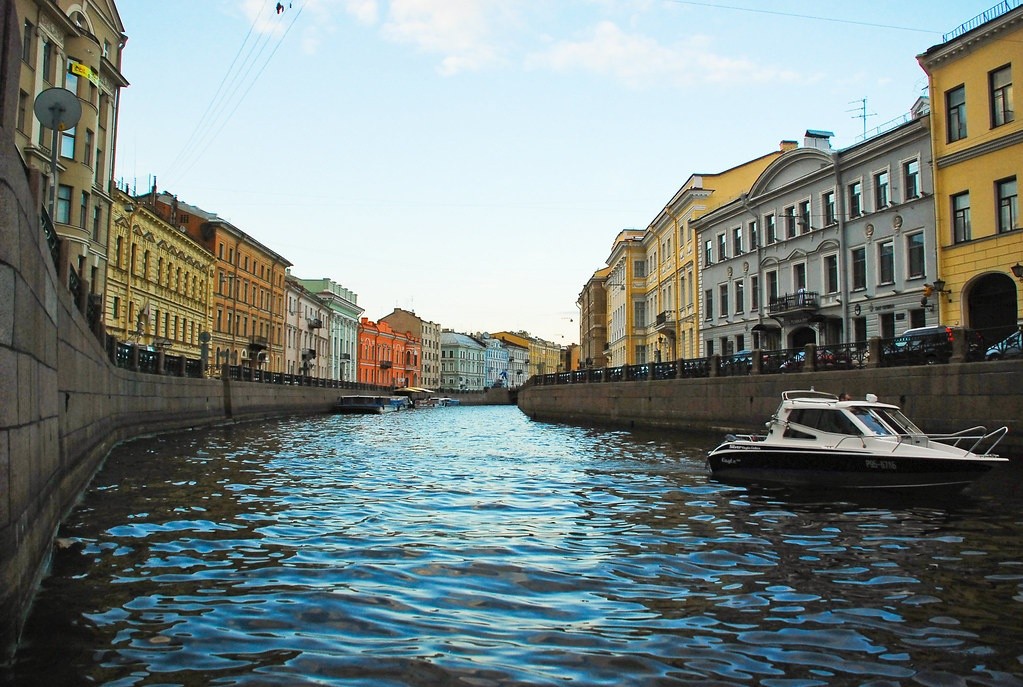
[985,331,1023,361]
[779,344,847,374]
[720,348,779,371]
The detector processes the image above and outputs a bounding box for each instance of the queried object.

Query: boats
[335,387,461,415]
[704,386,1009,503]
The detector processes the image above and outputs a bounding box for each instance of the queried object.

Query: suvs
[882,325,955,367]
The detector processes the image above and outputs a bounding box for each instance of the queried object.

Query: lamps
[621,285,625,290]
[570,319,573,322]
[920,297,934,312]
[933,279,952,303]
[125,205,133,212]
[1011,262,1023,283]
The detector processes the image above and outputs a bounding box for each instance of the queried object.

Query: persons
[831,393,878,435]
